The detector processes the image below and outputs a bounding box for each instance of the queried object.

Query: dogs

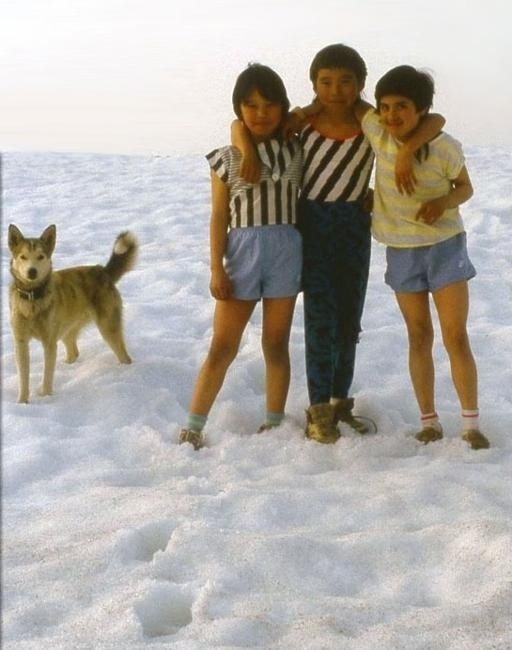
[6,221,140,404]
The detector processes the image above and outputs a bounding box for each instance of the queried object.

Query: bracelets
[292,105,307,121]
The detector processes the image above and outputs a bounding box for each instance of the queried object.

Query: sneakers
[462,431,489,448]
[179,429,203,450]
[305,404,340,443]
[330,398,370,434]
[415,429,442,444]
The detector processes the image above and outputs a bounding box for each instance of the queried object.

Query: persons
[229,44,446,444]
[281,64,494,451]
[177,62,374,449]
